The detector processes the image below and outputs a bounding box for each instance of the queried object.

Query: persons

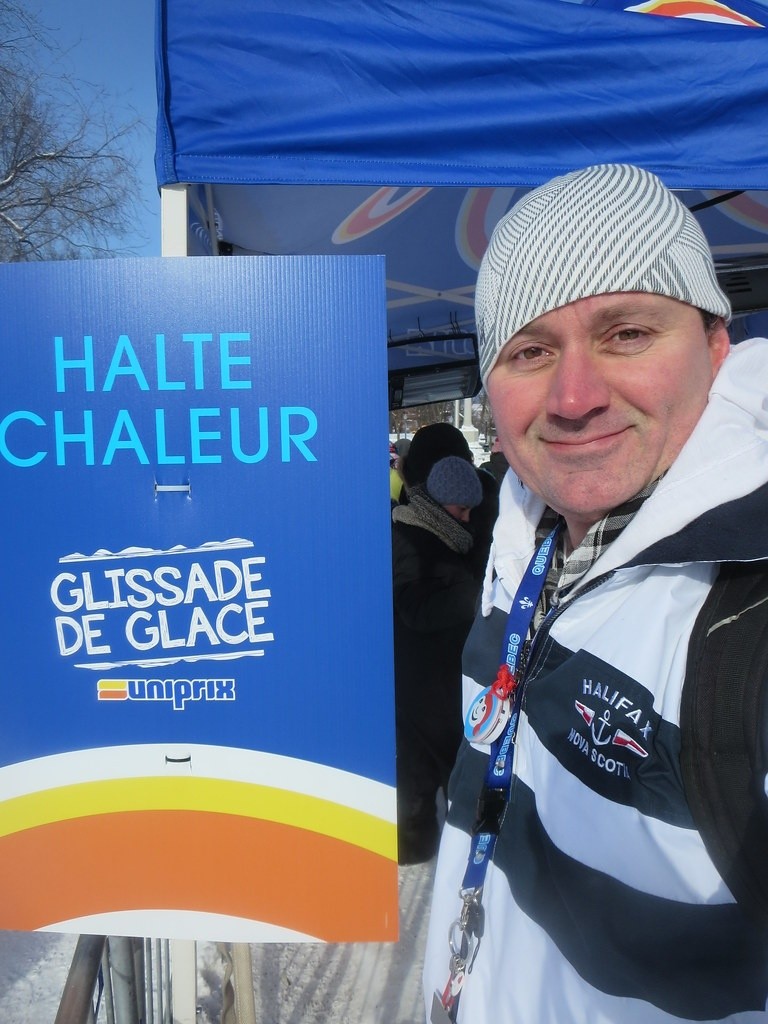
[391,456,484,867]
[389,424,510,586]
[424,165,767,1024]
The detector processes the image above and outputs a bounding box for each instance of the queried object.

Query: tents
[157,0,768,1024]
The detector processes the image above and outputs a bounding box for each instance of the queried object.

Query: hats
[473,163,732,389]
[426,456,482,505]
